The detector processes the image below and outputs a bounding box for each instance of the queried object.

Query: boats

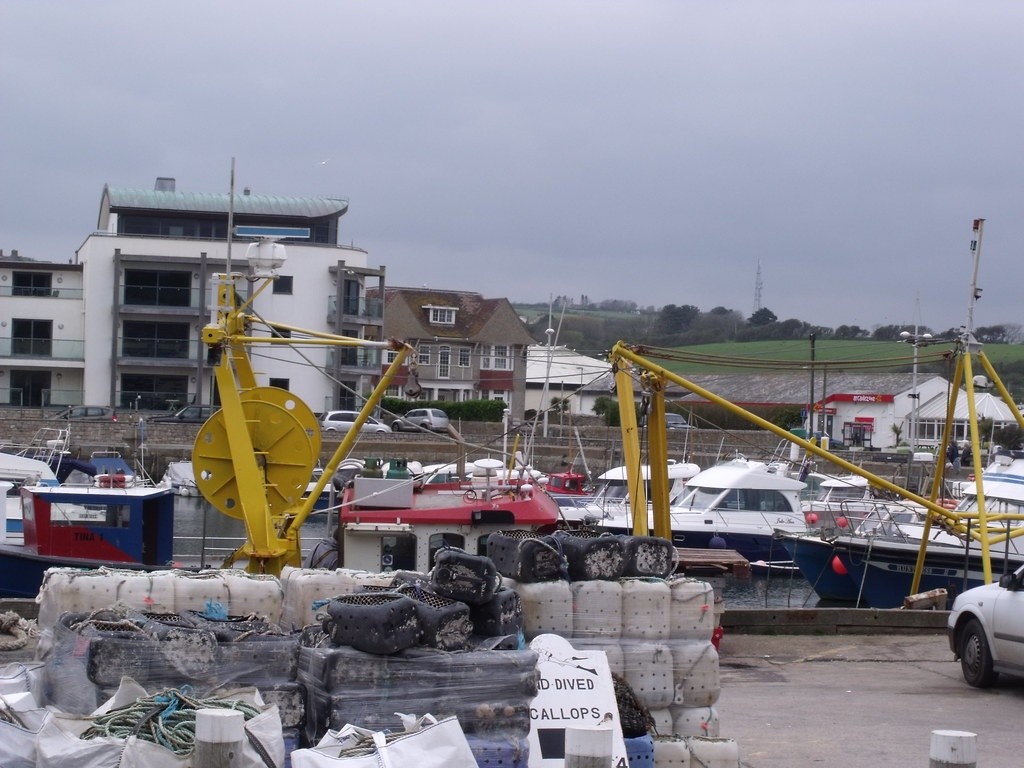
[0,407,871,603]
[833,416,1024,608]
[772,498,963,600]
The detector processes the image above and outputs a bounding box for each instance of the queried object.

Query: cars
[665,412,696,429]
[49,405,118,421]
[318,410,393,433]
[390,408,450,433]
[146,405,221,422]
[948,564,1023,690]
[813,431,843,449]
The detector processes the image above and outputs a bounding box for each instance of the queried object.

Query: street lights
[543,293,555,439]
[900,298,932,452]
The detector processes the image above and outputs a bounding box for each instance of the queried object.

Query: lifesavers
[94,482,133,488]
[94,473,133,480]
[935,498,958,509]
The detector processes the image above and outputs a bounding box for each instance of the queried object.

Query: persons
[960,443,971,466]
[947,441,959,466]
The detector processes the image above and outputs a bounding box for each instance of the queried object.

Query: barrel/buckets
[0,529,740,768]
